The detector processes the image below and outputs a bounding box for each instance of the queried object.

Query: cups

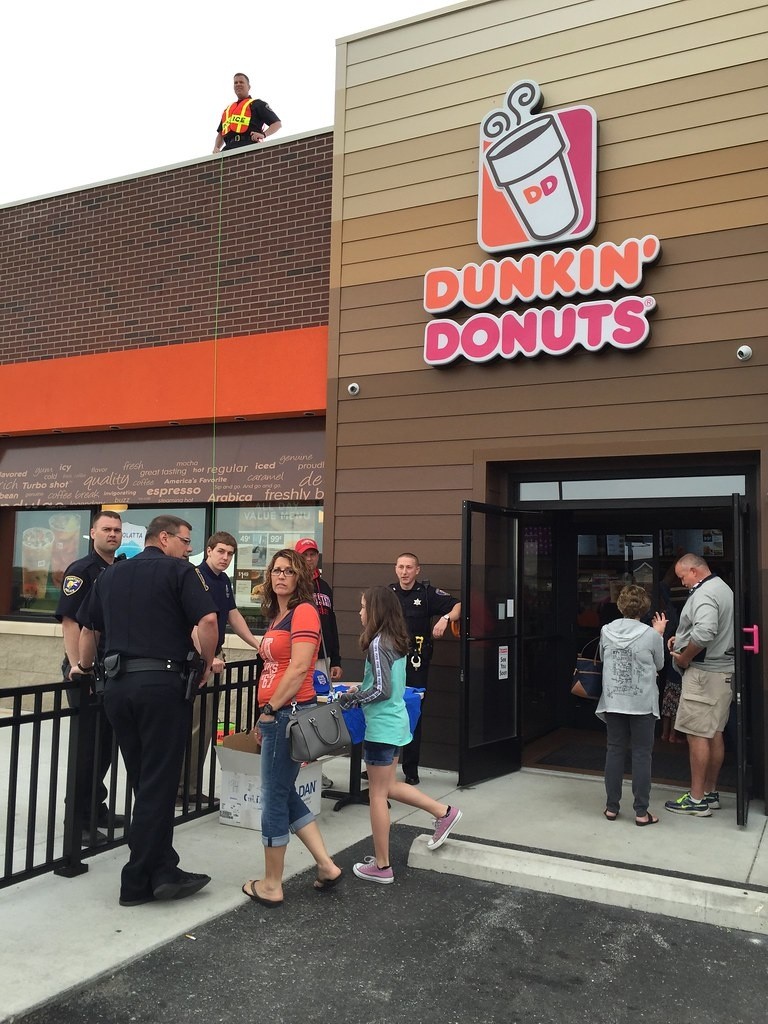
[50,513,81,587]
[22,527,55,599]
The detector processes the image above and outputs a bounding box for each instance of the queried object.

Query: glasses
[160,530,191,545]
[270,567,296,577]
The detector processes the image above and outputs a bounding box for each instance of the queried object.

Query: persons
[55,511,127,848]
[361,552,462,786]
[241,549,343,909]
[68,515,219,906]
[293,538,343,681]
[174,531,261,811]
[213,73,282,153]
[595,585,670,827]
[665,552,735,817]
[337,584,462,885]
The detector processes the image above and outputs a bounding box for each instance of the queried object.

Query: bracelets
[77,660,94,673]
[262,131,266,138]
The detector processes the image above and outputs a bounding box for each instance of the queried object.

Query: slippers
[242,880,281,909]
[635,811,659,826]
[313,869,344,890]
[604,809,618,820]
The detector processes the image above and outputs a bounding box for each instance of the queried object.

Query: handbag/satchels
[572,637,602,699]
[286,702,351,762]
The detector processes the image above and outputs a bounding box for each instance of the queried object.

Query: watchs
[262,703,277,717]
[444,613,450,625]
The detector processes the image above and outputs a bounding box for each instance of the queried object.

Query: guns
[183,650,208,704]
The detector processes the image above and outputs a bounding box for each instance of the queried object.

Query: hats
[296,539,319,555]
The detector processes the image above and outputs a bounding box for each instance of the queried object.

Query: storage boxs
[213,733,322,833]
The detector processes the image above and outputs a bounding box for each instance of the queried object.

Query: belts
[126,658,183,672]
[226,135,251,146]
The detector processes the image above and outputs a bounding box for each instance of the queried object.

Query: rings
[254,732,258,735]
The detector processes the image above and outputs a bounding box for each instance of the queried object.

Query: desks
[317,681,425,813]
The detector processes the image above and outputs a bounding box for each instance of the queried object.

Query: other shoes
[405,774,420,785]
[360,771,368,780]
[154,867,211,900]
[119,893,155,906]
[661,736,689,744]
[98,813,125,828]
[81,827,106,846]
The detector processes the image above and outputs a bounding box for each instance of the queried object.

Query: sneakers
[353,856,394,884]
[322,775,333,789]
[175,794,220,810]
[665,792,720,817]
[427,804,463,851]
